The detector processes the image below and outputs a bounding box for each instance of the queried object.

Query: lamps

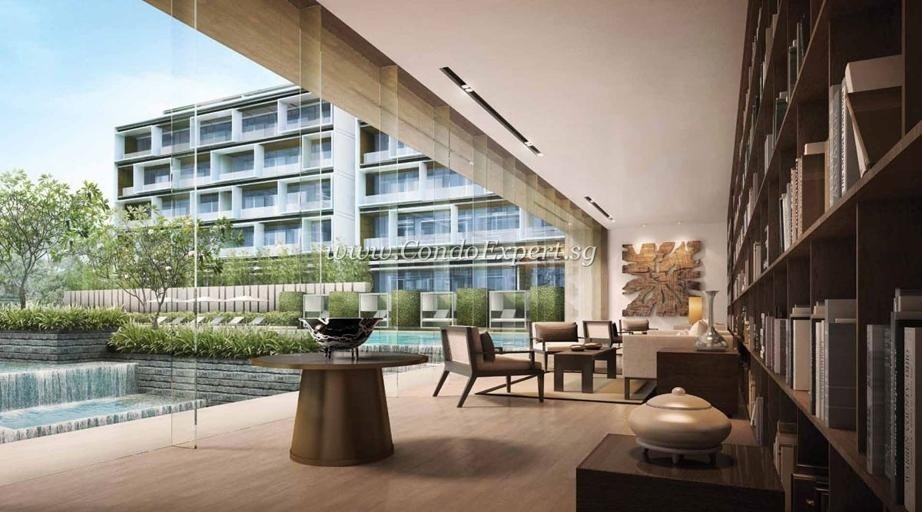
[688,296,703,324]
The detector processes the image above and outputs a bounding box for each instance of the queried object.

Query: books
[726,3,921,512]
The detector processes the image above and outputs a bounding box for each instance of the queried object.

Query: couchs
[622,324,733,400]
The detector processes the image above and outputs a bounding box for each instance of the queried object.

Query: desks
[576,433,786,512]
[250,351,428,468]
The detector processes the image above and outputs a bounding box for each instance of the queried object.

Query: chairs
[433,318,658,409]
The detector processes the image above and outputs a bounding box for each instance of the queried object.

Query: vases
[695,290,729,351]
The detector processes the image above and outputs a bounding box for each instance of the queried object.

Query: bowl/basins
[297,315,384,351]
